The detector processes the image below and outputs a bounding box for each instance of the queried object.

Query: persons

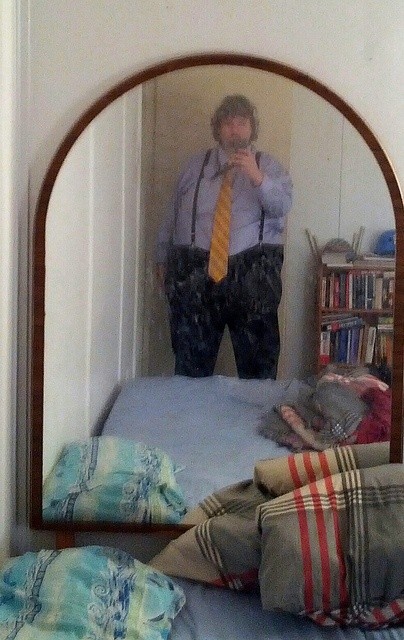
[153,91,294,381]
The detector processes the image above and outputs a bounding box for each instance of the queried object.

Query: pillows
[43,434,190,524]
[2,546,186,639]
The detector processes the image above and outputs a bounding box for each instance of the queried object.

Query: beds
[1,539,403,638]
[75,372,391,521]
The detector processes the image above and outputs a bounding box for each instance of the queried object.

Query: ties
[208,150,237,284]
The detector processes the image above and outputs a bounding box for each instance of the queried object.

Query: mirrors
[31,49,404,537]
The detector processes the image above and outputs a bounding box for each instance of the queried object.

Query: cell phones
[234,138,247,153]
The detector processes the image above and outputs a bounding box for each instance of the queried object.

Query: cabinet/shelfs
[312,255,397,377]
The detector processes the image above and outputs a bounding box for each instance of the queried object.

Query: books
[318,311,394,376]
[315,253,396,311]
